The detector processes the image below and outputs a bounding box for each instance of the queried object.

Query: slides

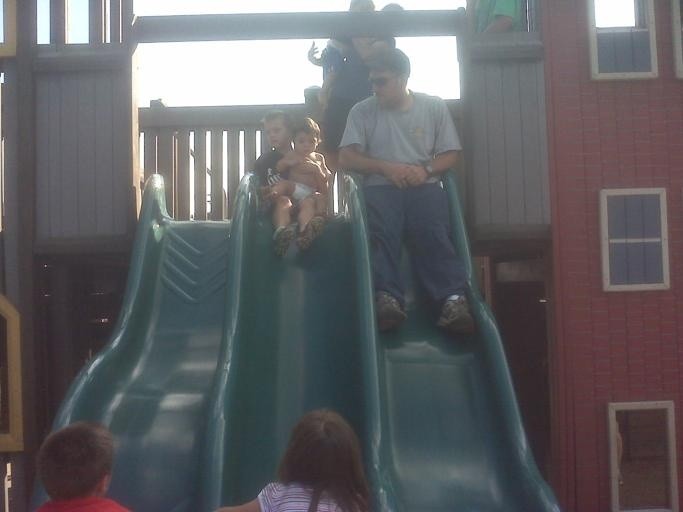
[31,174,561,512]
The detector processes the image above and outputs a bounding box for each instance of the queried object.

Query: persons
[465,0,522,35]
[338,48,474,335]
[255,110,332,258]
[213,409,378,512]
[31,420,135,512]
[308,1,403,155]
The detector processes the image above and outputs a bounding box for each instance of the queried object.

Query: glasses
[367,76,389,86]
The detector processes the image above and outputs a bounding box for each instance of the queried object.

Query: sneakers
[436,297,473,332]
[272,216,327,260]
[376,292,407,332]
